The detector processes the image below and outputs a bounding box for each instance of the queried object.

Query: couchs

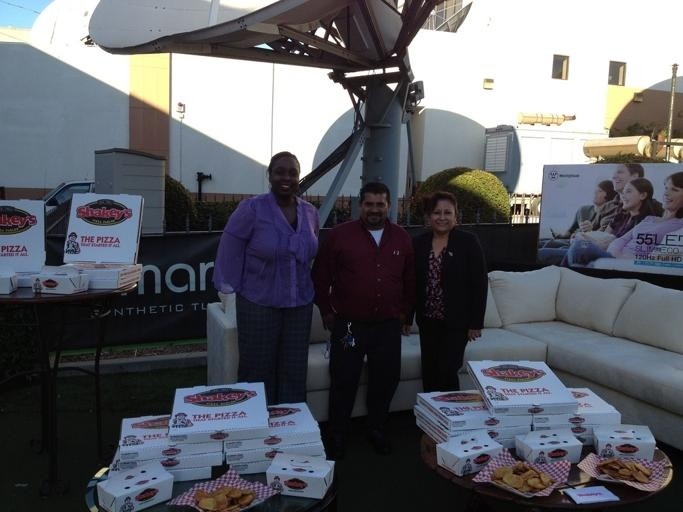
[206,264,682,451]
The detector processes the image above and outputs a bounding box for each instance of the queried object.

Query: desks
[0,282,140,470]
[64,408,673,512]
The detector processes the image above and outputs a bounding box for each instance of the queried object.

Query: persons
[552,180,616,240]
[214,151,319,407]
[543,164,644,246]
[559,171,683,269]
[537,177,664,267]
[402,191,488,392]
[311,183,416,460]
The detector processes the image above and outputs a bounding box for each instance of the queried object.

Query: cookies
[193,486,256,512]
[595,457,651,484]
[490,460,556,493]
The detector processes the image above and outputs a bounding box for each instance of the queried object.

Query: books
[564,485,619,504]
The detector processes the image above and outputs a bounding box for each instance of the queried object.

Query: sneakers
[328,436,346,459]
[370,430,389,455]
